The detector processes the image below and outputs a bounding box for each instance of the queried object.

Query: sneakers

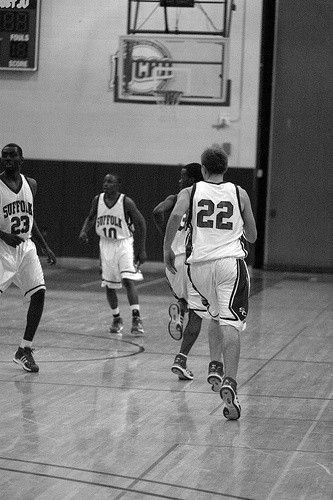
[109,316,124,332]
[131,315,144,334]
[170,358,194,380]
[12,345,39,372]
[168,302,184,340]
[207,363,225,392]
[220,378,241,419]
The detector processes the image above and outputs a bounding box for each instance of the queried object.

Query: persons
[162,147,257,420]
[152,163,204,382]
[0,143,58,374]
[79,171,147,338]
